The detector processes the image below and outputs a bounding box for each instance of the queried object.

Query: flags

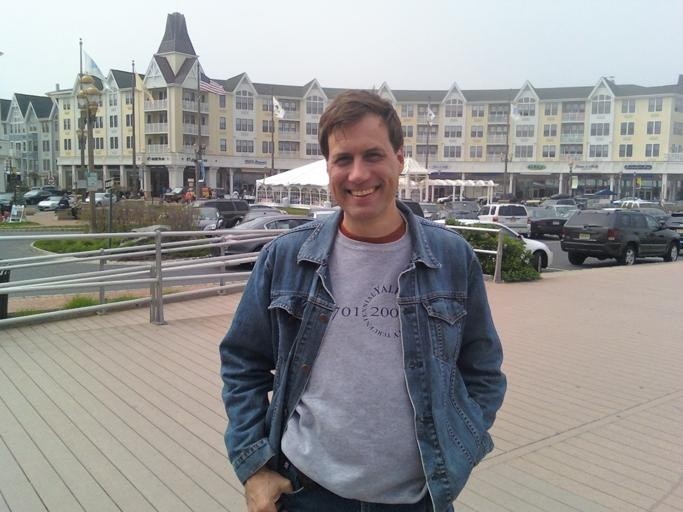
[199,72,226,96]
[272,95,286,119]
[426,103,436,127]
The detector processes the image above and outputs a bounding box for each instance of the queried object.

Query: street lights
[619,171,623,199]
[633,171,636,202]
[192,141,206,200]
[568,160,574,197]
[74,75,104,230]
[499,151,514,196]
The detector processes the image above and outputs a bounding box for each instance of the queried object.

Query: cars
[426,216,555,277]
[209,214,321,272]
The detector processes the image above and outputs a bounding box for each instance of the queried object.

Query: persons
[216,88,506,511]
[53,190,90,220]
[160,185,248,204]
[115,188,143,202]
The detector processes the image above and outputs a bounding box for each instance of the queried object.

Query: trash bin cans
[0,269,10,319]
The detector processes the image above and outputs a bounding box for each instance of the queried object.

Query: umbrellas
[594,187,616,199]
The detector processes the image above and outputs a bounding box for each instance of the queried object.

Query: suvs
[560,206,682,268]
